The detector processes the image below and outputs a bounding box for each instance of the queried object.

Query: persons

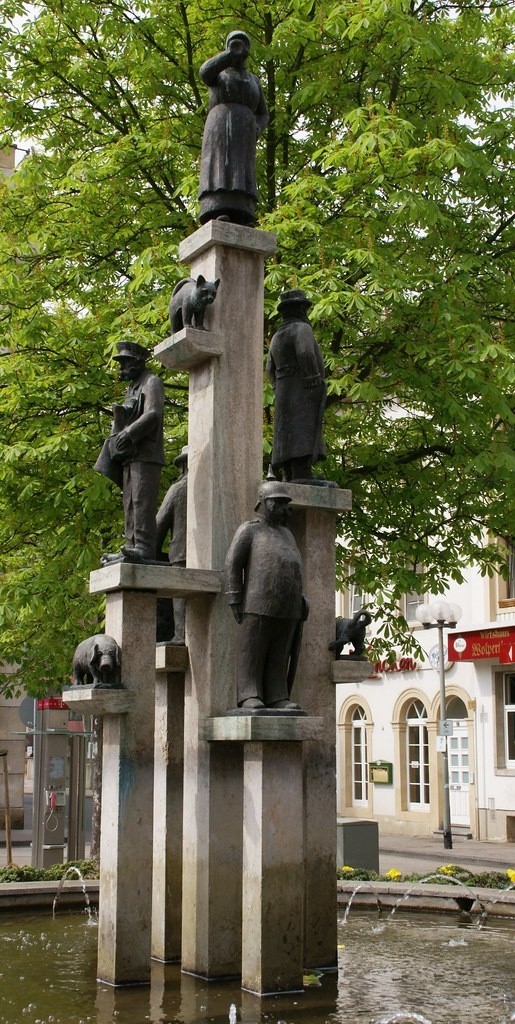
[266,290,329,478]
[198,30,269,228]
[223,478,308,708]
[155,445,187,646]
[94,342,166,560]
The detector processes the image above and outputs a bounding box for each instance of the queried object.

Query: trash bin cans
[337,819,380,877]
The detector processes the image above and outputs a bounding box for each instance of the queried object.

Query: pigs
[72,634,122,684]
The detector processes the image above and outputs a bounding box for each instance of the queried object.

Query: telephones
[45,790,66,808]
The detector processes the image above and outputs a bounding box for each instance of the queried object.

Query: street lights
[415,601,462,849]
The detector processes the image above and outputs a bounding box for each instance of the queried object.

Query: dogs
[328,608,372,656]
